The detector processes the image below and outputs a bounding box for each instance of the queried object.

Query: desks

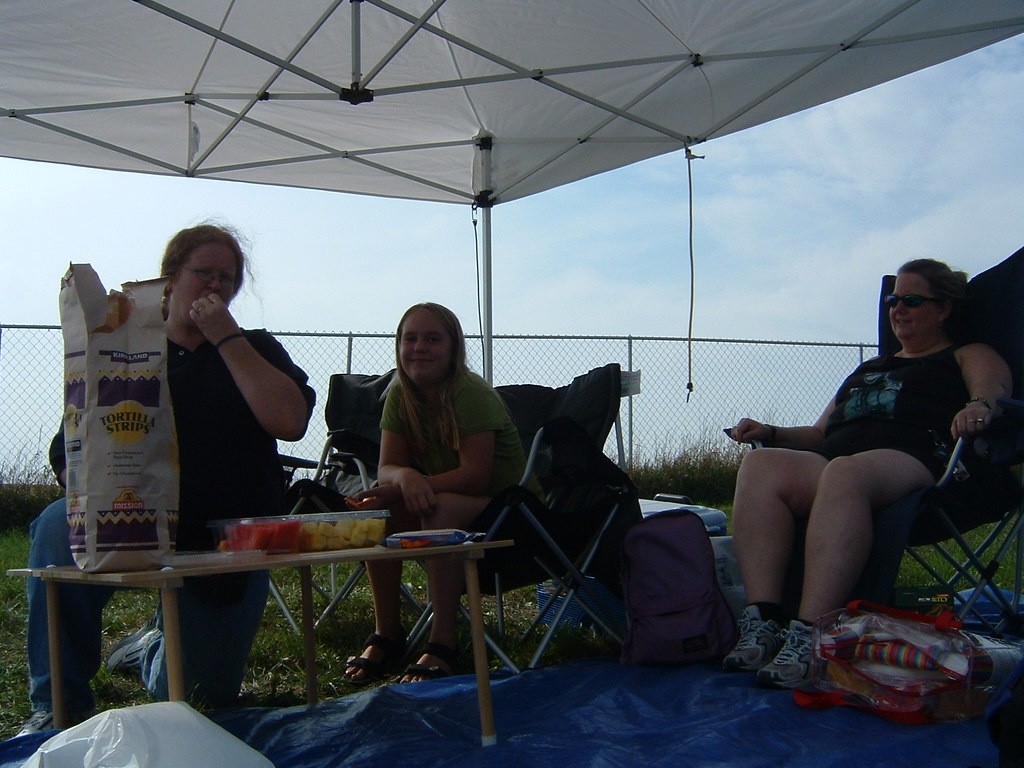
[5,540,514,748]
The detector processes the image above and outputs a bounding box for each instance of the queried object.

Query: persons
[6,225,316,743]
[344,303,523,684]
[724,259,1013,689]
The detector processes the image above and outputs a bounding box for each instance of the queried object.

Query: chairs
[722,247,1024,641]
[400,363,643,674]
[269,369,424,639]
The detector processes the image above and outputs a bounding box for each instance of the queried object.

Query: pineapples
[303,517,385,550]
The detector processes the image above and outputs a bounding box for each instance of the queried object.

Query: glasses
[881,293,947,308]
[185,264,242,291]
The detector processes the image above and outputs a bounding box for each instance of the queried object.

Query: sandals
[342,631,409,686]
[399,648,461,684]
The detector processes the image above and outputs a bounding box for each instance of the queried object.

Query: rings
[976,419,984,422]
[967,420,976,423]
[197,306,205,313]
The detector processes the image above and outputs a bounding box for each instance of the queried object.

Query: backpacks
[618,510,741,669]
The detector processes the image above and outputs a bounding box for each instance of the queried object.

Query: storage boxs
[895,584,954,610]
[535,498,728,634]
[206,510,392,555]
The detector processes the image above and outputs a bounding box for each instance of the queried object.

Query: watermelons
[224,522,301,554]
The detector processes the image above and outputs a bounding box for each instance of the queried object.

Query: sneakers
[754,619,837,690]
[105,616,164,674]
[721,606,783,671]
[12,688,97,743]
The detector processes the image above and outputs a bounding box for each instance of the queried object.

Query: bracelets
[423,473,437,494]
[764,424,777,438]
[965,398,993,410]
[214,333,244,351]
